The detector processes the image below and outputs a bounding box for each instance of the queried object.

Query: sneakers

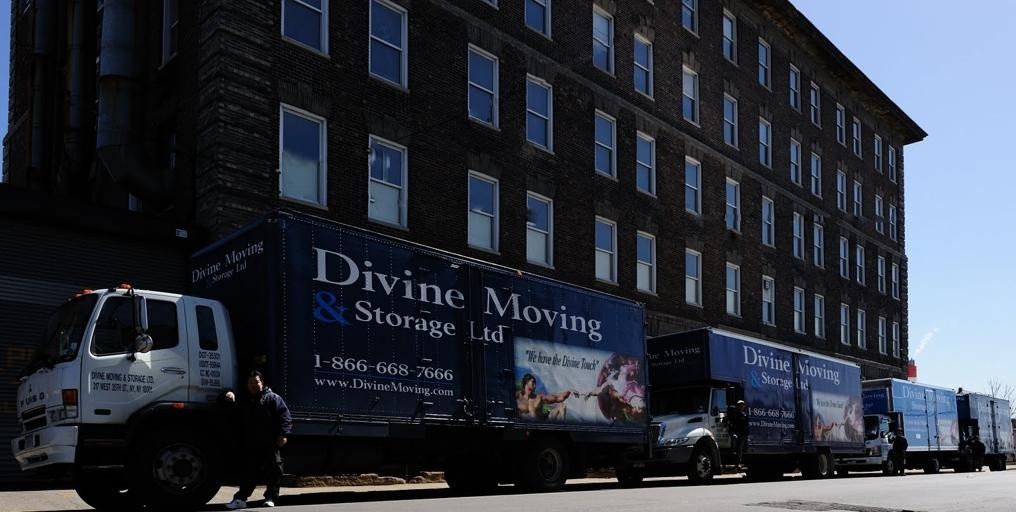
[225,499,246,509]
[263,498,274,507]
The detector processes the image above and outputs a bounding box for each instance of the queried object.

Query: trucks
[957,390,1014,476]
[638,320,871,484]
[11,203,666,510]
[832,372,963,479]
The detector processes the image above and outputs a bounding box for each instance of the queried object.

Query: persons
[815,414,838,441]
[581,359,646,410]
[516,374,581,421]
[838,399,864,435]
[889,427,908,476]
[217,370,294,509]
[960,432,985,472]
